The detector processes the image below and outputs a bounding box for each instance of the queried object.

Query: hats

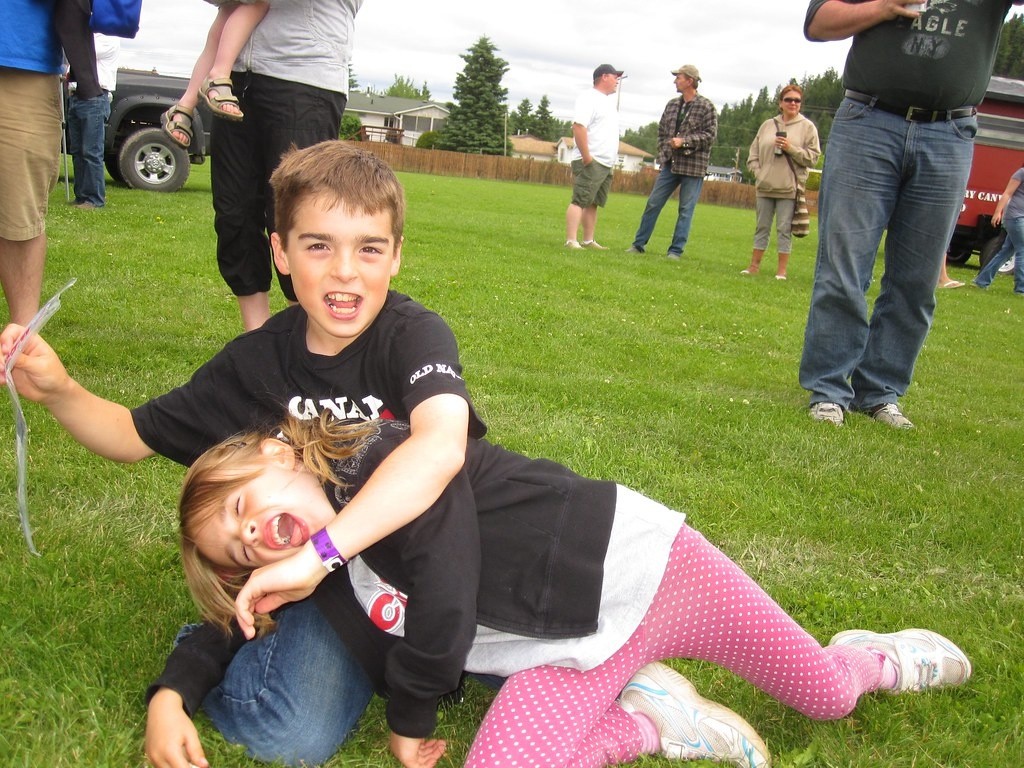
[672,64,702,83]
[593,64,624,77]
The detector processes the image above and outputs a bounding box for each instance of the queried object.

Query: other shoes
[564,241,584,249]
[70,201,97,210]
[970,283,978,288]
[775,272,787,280]
[667,252,681,259]
[581,241,609,250]
[741,268,759,275]
[808,402,844,427]
[624,245,640,252]
[871,403,915,430]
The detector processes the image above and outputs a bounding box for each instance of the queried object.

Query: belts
[844,90,973,123]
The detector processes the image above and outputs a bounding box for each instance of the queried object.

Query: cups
[775,132,787,156]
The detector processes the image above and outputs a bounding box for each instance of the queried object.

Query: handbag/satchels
[792,180,810,237]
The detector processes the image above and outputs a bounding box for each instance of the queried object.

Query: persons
[970,165,1024,295]
[937,254,965,288]
[160,0,270,150]
[0,140,488,768]
[210,0,363,333]
[739,85,821,281]
[624,65,718,261]
[798,0,1024,428]
[0,0,142,328]
[564,64,623,250]
[64,33,120,210]
[142,407,972,768]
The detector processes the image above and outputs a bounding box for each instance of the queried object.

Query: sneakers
[829,628,972,694]
[620,662,771,768]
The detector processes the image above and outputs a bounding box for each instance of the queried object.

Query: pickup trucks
[64,67,212,191]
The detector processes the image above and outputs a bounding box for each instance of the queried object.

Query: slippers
[938,281,965,288]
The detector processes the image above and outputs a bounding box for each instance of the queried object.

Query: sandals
[197,77,244,121]
[160,105,194,149]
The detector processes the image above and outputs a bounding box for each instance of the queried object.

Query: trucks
[944,76,1024,275]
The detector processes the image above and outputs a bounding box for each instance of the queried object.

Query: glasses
[781,98,802,103]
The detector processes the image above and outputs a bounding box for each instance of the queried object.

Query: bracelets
[309,526,348,573]
